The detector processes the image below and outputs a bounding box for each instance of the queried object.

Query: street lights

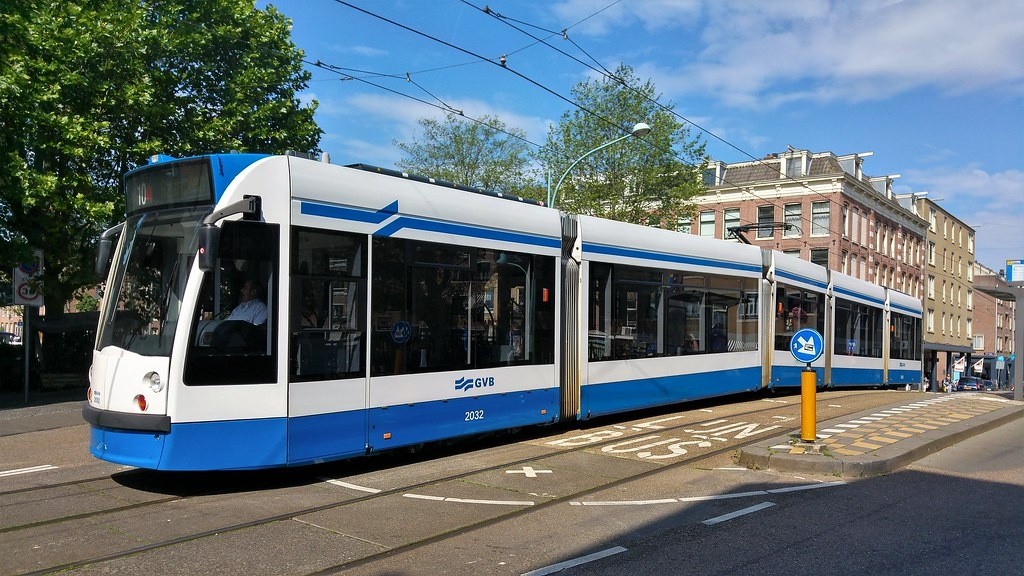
[546,121,653,209]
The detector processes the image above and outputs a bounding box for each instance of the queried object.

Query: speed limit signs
[18,284,39,300]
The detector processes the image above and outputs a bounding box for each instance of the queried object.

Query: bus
[81,152,925,471]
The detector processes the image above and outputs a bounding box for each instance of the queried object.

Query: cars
[983,379,999,391]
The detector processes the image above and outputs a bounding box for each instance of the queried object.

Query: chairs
[238,276,276,353]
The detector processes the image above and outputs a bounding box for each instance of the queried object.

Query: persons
[205,269,270,345]
[942,372,954,394]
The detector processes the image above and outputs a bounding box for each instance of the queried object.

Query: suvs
[957,376,984,391]
